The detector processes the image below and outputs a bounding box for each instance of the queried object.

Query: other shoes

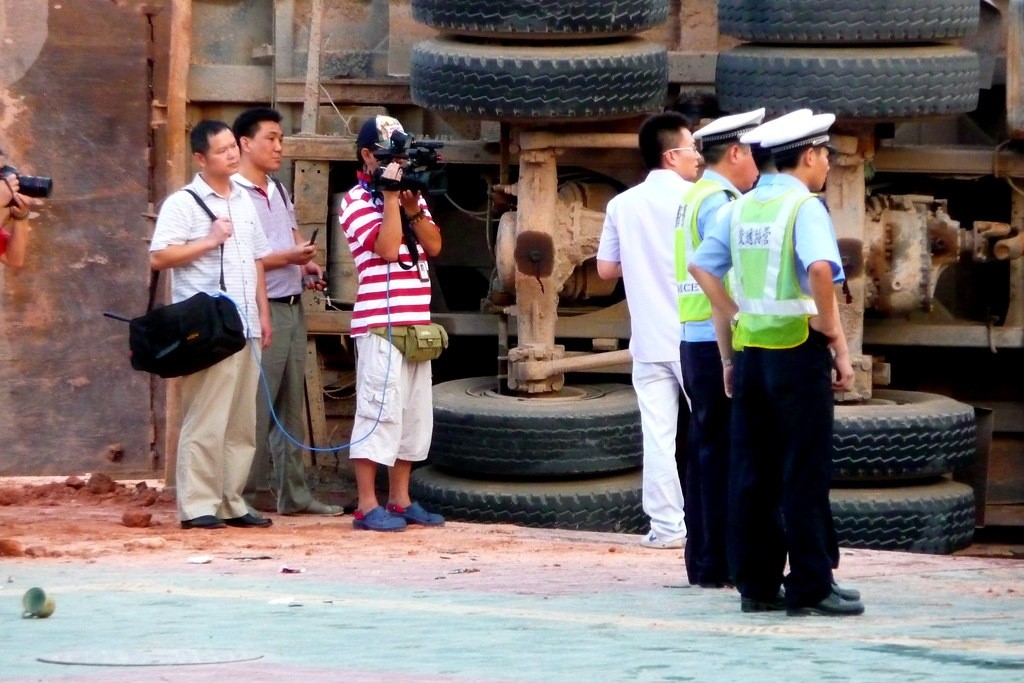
[296,498,345,515]
[244,503,263,518]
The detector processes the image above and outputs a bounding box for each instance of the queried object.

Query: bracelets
[12,213,29,220]
[408,209,425,224]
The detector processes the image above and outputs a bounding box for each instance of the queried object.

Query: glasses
[663,143,697,155]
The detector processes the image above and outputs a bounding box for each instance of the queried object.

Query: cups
[22,587,57,619]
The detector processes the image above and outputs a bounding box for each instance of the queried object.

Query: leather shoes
[180,515,227,528]
[224,513,272,527]
[741,589,786,612]
[830,582,861,600]
[787,594,864,616]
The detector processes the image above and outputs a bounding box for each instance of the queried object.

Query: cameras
[0,165,52,208]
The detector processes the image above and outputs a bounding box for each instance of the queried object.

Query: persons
[674,108,766,589]
[229,108,345,516]
[339,116,445,532]
[598,112,701,547]
[687,108,865,619]
[0,174,33,267]
[149,119,273,530]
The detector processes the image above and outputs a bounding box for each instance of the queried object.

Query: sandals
[385,501,445,527]
[354,505,407,532]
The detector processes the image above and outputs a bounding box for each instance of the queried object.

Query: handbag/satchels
[371,322,448,361]
[129,292,247,377]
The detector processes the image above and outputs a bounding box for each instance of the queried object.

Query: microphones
[413,142,445,148]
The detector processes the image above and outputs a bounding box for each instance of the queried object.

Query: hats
[354,114,408,150]
[739,108,813,151]
[760,112,836,153]
[692,108,766,151]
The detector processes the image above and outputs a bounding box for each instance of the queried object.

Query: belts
[267,293,302,306]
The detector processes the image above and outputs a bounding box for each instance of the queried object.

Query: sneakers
[640,529,682,549]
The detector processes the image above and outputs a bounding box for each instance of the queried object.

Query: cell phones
[303,271,329,287]
